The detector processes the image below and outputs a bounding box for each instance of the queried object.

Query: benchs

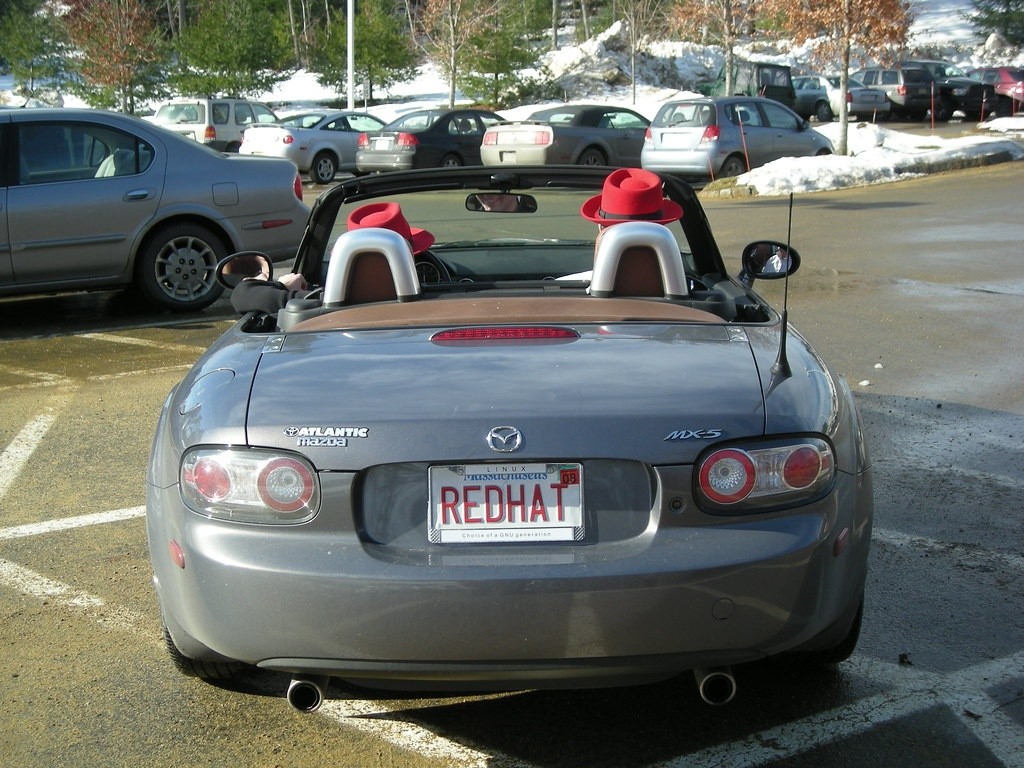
[94,147,136,178]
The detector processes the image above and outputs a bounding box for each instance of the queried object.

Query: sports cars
[146,164,876,716]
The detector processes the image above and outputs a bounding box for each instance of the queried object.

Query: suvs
[890,61,999,123]
[845,66,941,122]
[714,58,796,114]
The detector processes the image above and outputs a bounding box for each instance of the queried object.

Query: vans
[149,96,282,153]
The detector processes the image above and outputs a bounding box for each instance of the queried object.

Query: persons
[240,256,270,281]
[276,201,435,300]
[476,194,520,212]
[763,246,792,274]
[556,169,710,293]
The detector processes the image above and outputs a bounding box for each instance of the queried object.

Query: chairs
[461,119,472,135]
[590,220,691,299]
[674,113,685,122]
[235,110,249,123]
[322,227,423,305]
[740,110,752,127]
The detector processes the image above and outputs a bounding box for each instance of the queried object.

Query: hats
[580,167,682,226]
[347,202,435,255]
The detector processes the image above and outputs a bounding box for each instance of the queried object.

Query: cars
[356,106,510,171]
[0,106,312,308]
[237,108,391,183]
[638,96,838,188]
[479,104,652,168]
[786,73,891,124]
[961,65,1024,115]
